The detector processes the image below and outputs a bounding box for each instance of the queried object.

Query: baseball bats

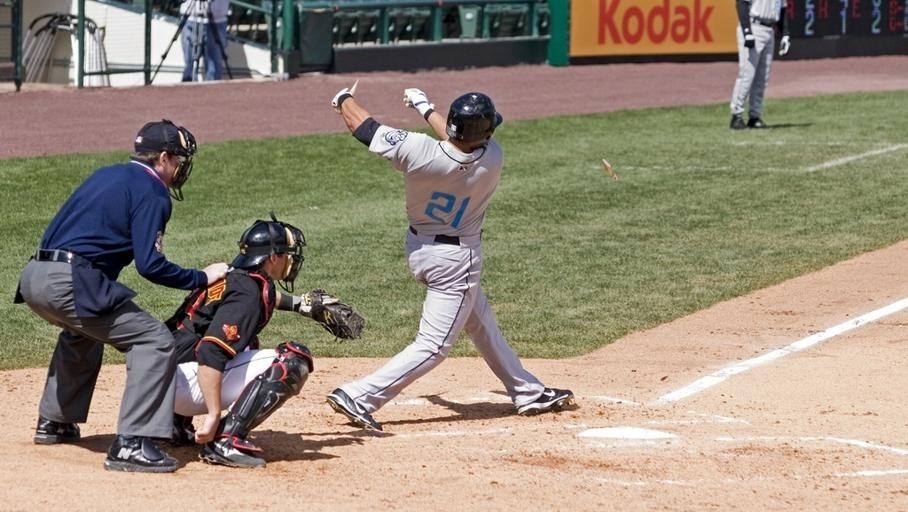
[334,80,360,113]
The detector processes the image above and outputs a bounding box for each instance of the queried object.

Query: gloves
[403,89,435,116]
[779,36,791,56]
[332,88,353,113]
[744,34,755,48]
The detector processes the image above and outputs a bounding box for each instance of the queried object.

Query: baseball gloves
[300,288,366,340]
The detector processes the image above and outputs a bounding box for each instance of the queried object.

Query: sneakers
[34,415,80,444]
[514,387,573,415]
[731,115,765,129]
[104,415,267,473]
[325,388,383,433]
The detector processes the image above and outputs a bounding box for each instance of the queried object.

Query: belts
[409,226,459,245]
[750,16,774,27]
[33,249,72,263]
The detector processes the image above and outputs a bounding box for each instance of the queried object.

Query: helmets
[135,119,197,200]
[446,92,502,139]
[232,217,307,292]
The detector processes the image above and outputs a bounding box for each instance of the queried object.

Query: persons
[15,117,227,475]
[329,78,575,433]
[727,0,793,130]
[180,0,229,81]
[166,212,367,470]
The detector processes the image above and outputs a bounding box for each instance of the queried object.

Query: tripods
[150,0,234,84]
